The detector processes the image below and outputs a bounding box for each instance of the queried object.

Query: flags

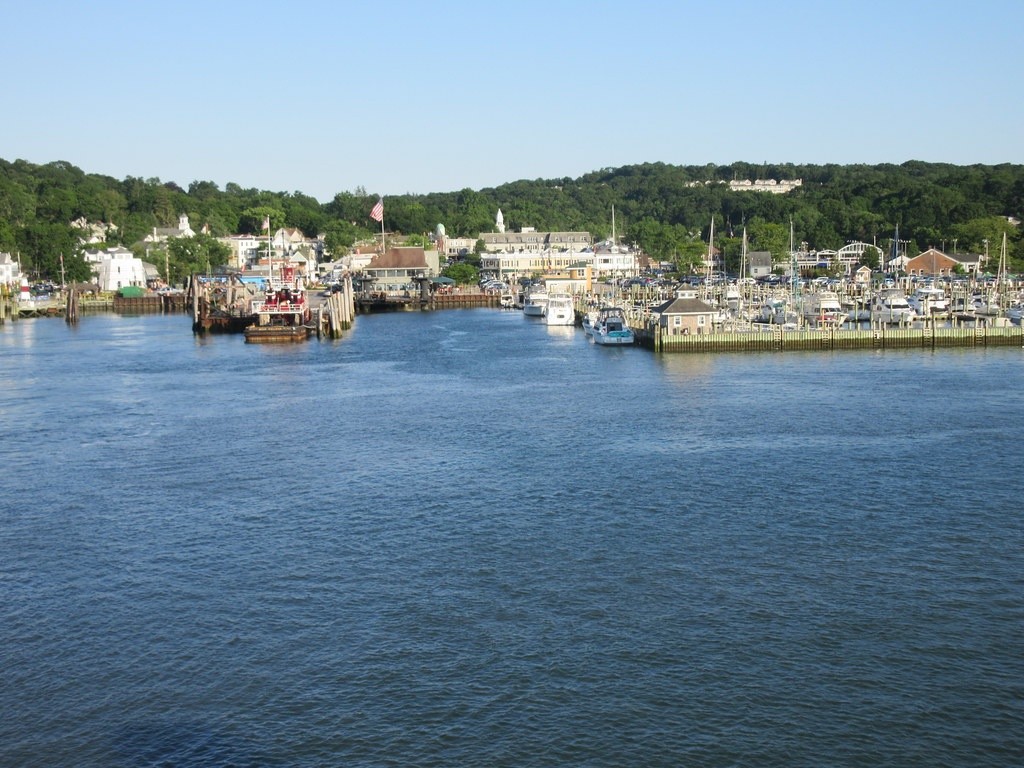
[261,216,269,230]
[370,198,384,221]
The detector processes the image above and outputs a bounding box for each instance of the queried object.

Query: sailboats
[705,215,1024,326]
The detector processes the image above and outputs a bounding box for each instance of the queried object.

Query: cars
[323,284,339,298]
[604,273,704,287]
[591,276,597,283]
[479,277,508,296]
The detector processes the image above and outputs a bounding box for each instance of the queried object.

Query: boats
[524,285,549,316]
[500,295,515,308]
[592,307,634,346]
[544,292,577,326]
[581,311,598,335]
